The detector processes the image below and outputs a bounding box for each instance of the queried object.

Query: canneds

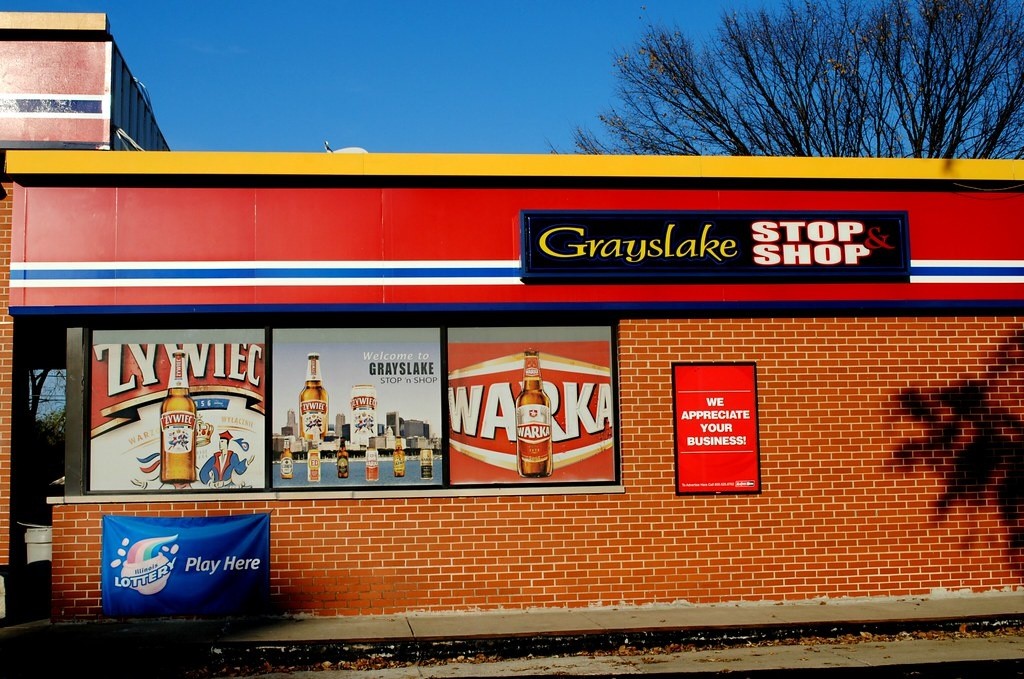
[350,384,378,445]
[365,447,379,482]
[420,448,433,480]
[308,449,321,482]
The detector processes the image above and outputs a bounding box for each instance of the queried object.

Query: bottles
[515,347,552,478]
[160,351,198,483]
[280,439,294,478]
[336,437,349,478]
[299,353,328,443]
[393,436,406,476]
